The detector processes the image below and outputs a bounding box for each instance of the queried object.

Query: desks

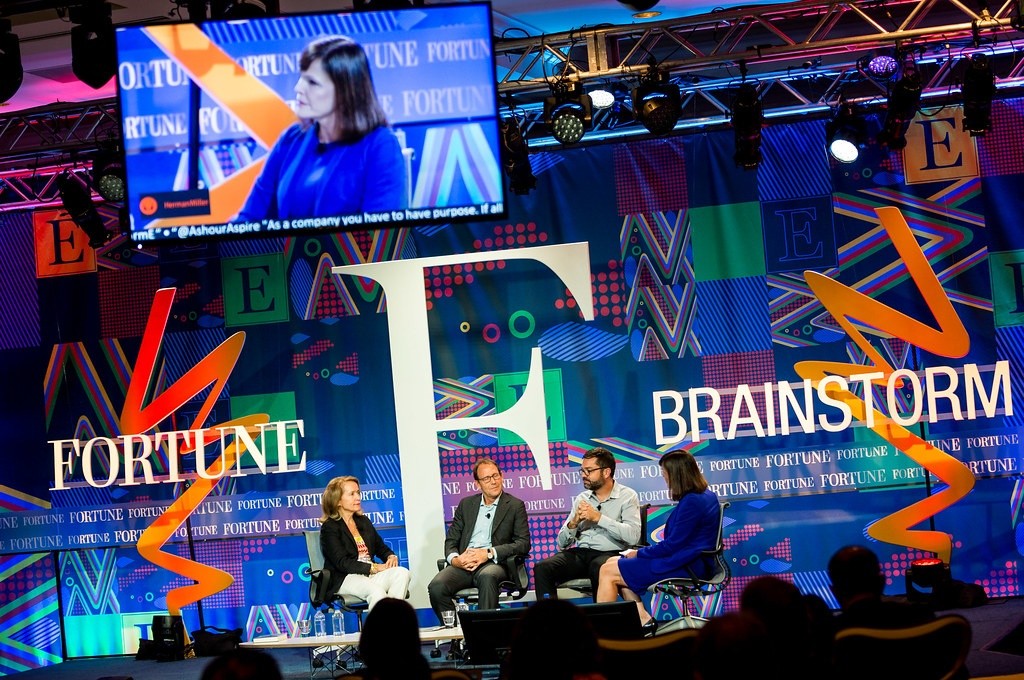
[240,626,464,680]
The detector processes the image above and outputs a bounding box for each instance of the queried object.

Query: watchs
[487,549,492,559]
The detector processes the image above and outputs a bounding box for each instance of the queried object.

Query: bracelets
[388,555,397,558]
[371,563,377,574]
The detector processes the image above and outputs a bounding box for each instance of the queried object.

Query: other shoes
[446,641,460,661]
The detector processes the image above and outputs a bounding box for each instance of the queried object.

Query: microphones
[316,142,326,152]
[487,513,490,518]
[597,505,601,510]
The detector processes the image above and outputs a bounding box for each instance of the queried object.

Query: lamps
[905,557,953,611]
[0,1,118,105]
[152,615,186,661]
[0,0,1005,248]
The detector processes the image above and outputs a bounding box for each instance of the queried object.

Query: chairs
[301,531,410,633]
[834,616,973,680]
[633,502,730,638]
[597,631,700,680]
[436,519,529,605]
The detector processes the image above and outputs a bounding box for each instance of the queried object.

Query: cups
[296,620,312,637]
[441,611,455,627]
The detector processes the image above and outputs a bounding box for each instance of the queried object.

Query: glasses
[578,467,609,476]
[477,473,501,484]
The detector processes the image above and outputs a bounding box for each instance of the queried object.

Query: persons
[533,447,641,604]
[318,476,411,611]
[428,459,531,660]
[357,598,432,680]
[697,576,836,680]
[510,598,608,680]
[227,34,410,223]
[827,546,970,680]
[597,449,720,637]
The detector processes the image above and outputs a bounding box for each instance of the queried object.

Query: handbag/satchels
[193,624,242,657]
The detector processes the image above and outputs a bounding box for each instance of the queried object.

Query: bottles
[314,607,327,636]
[456,599,468,628]
[332,607,344,636]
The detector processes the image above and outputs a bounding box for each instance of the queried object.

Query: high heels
[642,615,659,638]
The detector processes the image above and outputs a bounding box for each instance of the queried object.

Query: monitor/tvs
[111,0,509,245]
[457,601,645,668]
